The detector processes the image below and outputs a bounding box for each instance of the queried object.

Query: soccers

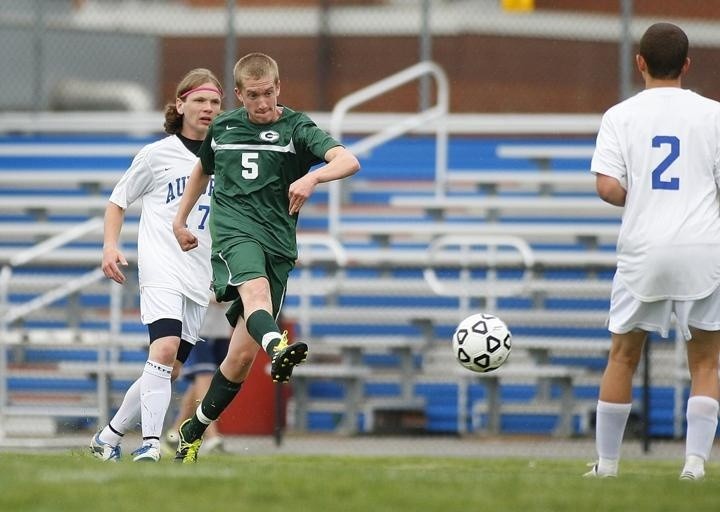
[452,312,514,372]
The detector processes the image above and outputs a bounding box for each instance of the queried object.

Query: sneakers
[89,430,123,464]
[583,463,617,479]
[679,470,705,481]
[271,330,308,385]
[131,445,161,463]
[172,419,204,463]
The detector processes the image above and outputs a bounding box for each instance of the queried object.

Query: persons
[581,23,720,482]
[89,67,222,463]
[173,52,361,463]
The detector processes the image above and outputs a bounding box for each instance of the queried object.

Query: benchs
[1,128,709,440]
